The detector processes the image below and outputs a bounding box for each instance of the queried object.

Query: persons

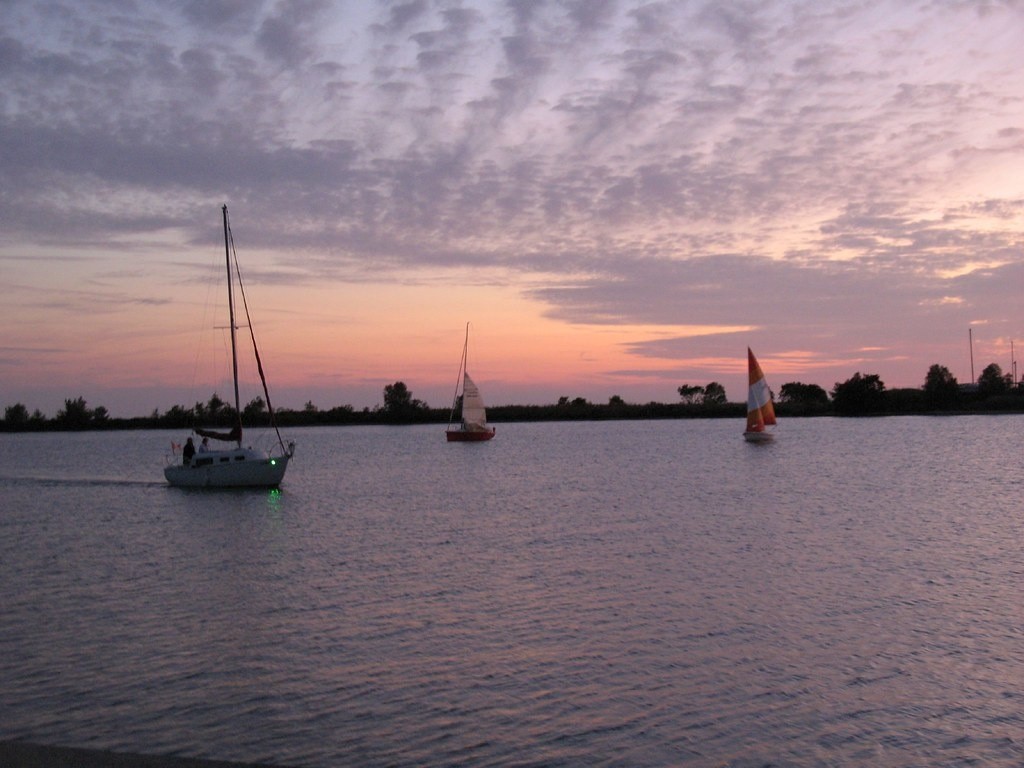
[183,437,196,470]
[198,437,210,453]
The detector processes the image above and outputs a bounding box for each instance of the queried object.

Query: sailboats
[165,201,297,489]
[741,347,778,444]
[444,322,495,443]
